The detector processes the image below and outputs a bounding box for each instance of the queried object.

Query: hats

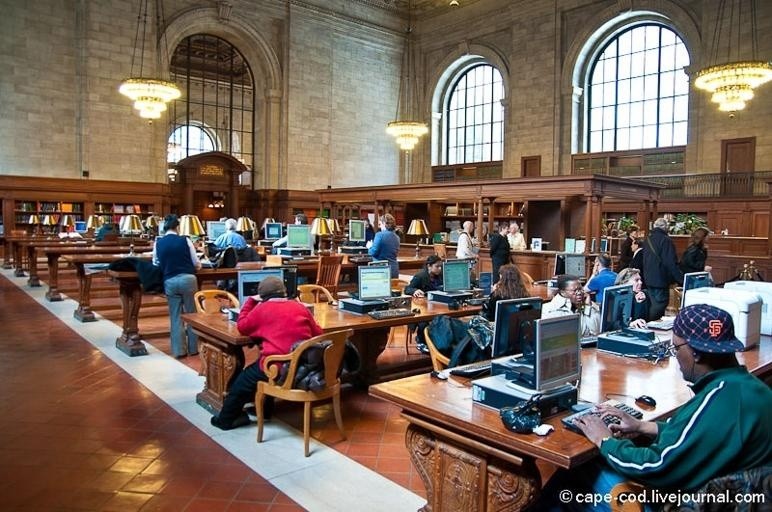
[674,304,745,354]
[599,251,610,267]
[259,276,287,300]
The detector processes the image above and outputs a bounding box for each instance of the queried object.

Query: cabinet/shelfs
[93,192,158,236]
[275,191,405,238]
[11,191,91,235]
[320,189,409,243]
[428,198,528,248]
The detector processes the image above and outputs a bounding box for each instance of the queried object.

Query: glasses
[667,342,687,353]
[564,289,584,295]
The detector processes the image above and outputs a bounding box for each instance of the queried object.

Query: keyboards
[560,398,643,436]
[580,337,598,346]
[368,309,414,320]
[449,360,491,378]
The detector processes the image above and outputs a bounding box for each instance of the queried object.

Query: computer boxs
[340,248,368,254]
[338,297,388,314]
[277,248,311,255]
[258,240,275,246]
[229,308,239,321]
[548,280,558,287]
[684,286,763,352]
[470,374,578,420]
[725,281,772,335]
[597,330,670,358]
[491,354,537,376]
[427,291,473,303]
[304,303,314,315]
[389,295,411,308]
[338,246,367,255]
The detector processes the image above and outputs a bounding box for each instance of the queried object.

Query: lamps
[407,219,429,261]
[388,1,428,155]
[42,214,56,230]
[28,214,39,233]
[118,1,181,128]
[123,214,144,254]
[311,215,331,257]
[146,217,158,239]
[236,215,251,243]
[325,218,340,252]
[692,1,771,118]
[449,0,459,8]
[59,214,74,232]
[85,215,102,236]
[178,214,205,251]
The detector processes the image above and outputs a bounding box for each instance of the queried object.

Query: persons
[272,214,309,248]
[541,275,600,337]
[211,276,324,430]
[507,223,527,250]
[486,264,530,322]
[617,226,638,273]
[366,213,400,279]
[615,268,647,329]
[631,238,644,271]
[214,218,246,249]
[489,222,510,285]
[456,221,480,261]
[406,255,443,354]
[679,228,709,273]
[573,303,772,511]
[363,218,375,241]
[643,218,684,320]
[152,214,202,359]
[583,253,617,303]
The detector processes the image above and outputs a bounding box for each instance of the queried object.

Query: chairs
[423,316,486,374]
[311,254,343,302]
[194,290,241,315]
[368,301,772,512]
[255,330,356,456]
[296,283,337,308]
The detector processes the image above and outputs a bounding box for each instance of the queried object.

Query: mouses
[636,395,656,408]
[412,308,420,313]
[637,323,646,328]
[418,294,424,298]
[536,423,555,435]
[431,370,448,380]
[220,305,229,314]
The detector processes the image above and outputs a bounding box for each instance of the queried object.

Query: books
[15,200,83,225]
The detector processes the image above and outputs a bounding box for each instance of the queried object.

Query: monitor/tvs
[208,222,225,240]
[565,254,586,277]
[287,224,311,250]
[353,265,392,303]
[680,272,708,310]
[443,261,470,295]
[554,254,565,276]
[491,296,543,369]
[343,220,365,248]
[265,223,282,240]
[600,284,655,341]
[158,221,164,235]
[261,265,298,298]
[74,221,88,234]
[506,313,581,396]
[238,270,283,310]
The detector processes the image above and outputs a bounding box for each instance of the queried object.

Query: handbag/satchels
[648,233,679,289]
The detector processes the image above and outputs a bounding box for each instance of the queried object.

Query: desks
[60,250,229,322]
[19,240,147,287]
[104,254,450,357]
[180,277,558,417]
[399,240,562,290]
[36,246,152,301]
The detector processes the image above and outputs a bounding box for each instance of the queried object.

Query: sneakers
[421,342,430,355]
[210,416,229,430]
